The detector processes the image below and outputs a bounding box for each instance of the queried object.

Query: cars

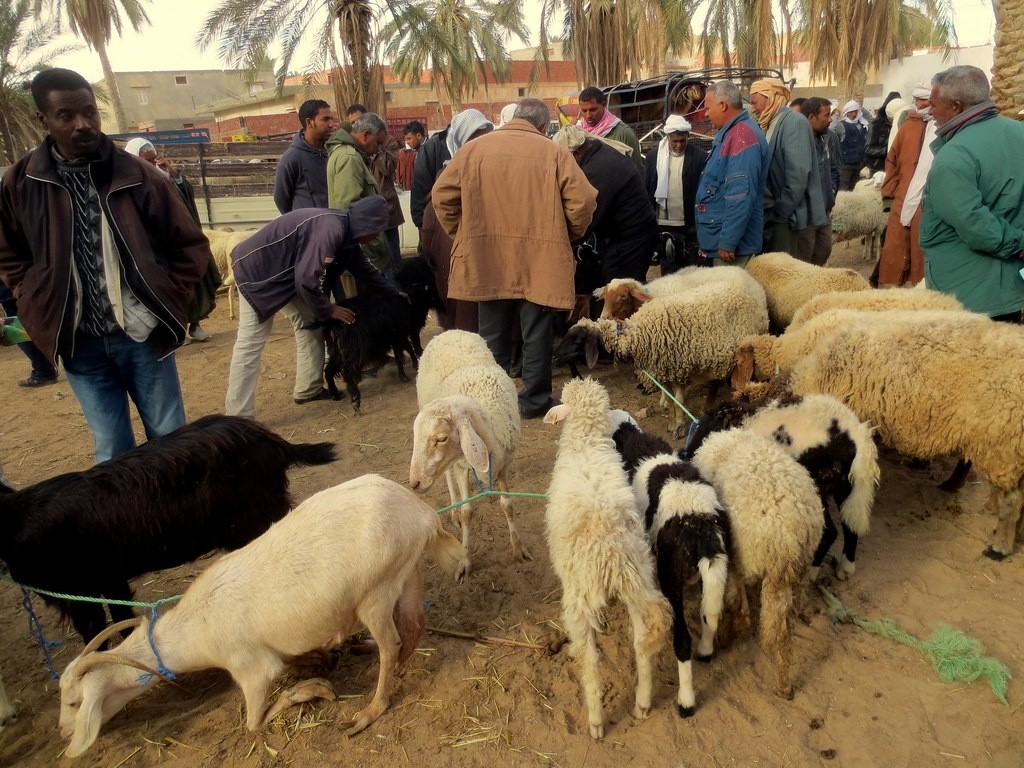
[545,120,560,140]
[199,158,250,185]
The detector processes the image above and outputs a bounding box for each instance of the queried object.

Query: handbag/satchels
[573,230,603,295]
[0,316,32,347]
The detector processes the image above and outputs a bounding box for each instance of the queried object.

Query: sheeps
[0,412,339,651]
[621,82,703,121]
[744,251,870,337]
[202,231,263,321]
[550,278,769,439]
[409,327,534,583]
[59,474,463,758]
[544,376,673,739]
[324,272,440,415]
[679,384,880,580]
[679,428,826,663]
[593,266,749,395]
[828,171,887,261]
[609,408,744,717]
[727,286,1024,560]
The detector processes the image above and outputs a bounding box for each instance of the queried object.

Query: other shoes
[183,337,192,346]
[901,457,933,474]
[294,388,345,405]
[508,341,524,378]
[597,347,618,365]
[17,376,59,387]
[520,397,563,419]
[185,323,209,342]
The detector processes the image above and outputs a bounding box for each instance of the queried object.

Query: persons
[225,195,411,422]
[919,64,1024,327]
[790,91,916,288]
[695,81,771,269]
[124,137,202,232]
[274,99,405,298]
[431,97,599,419]
[574,87,645,182]
[0,68,211,463]
[396,104,517,333]
[644,114,710,267]
[750,78,828,259]
[878,84,937,287]
[552,124,660,363]
[0,281,60,387]
